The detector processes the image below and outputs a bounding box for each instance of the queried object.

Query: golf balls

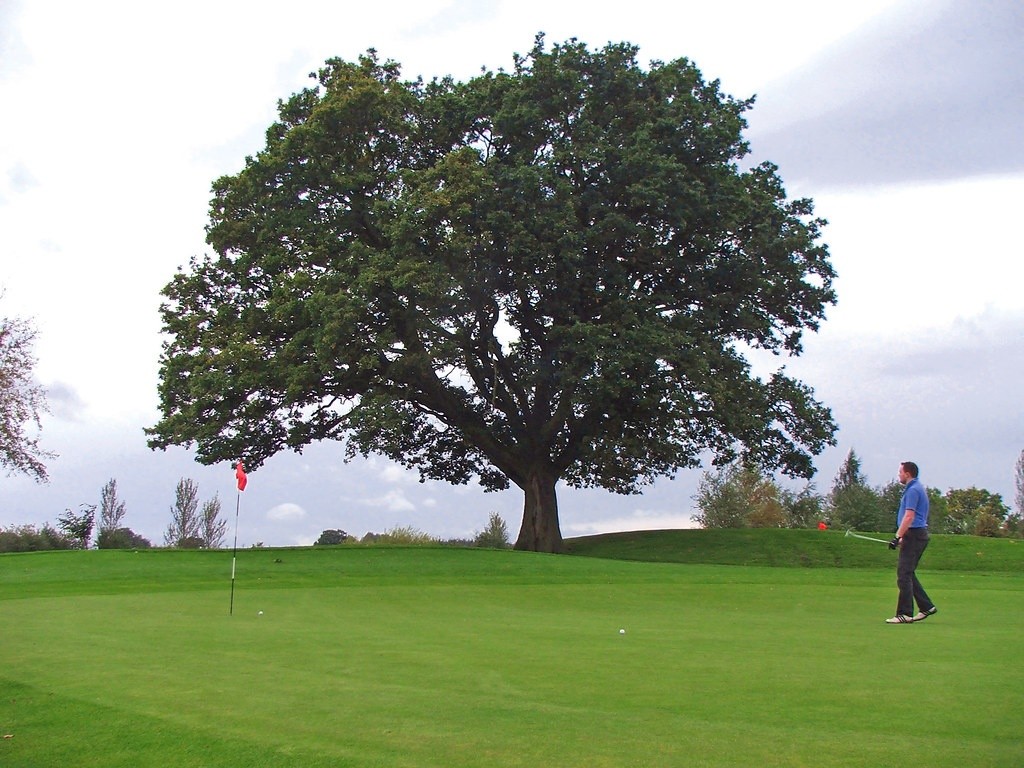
[259,611,263,615]
[620,629,626,633]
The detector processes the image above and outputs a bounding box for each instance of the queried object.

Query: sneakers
[886,614,913,623]
[913,606,937,621]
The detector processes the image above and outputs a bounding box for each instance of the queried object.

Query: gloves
[889,535,902,551]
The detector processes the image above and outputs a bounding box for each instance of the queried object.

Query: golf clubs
[845,528,903,547]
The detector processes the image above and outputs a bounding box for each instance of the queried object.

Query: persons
[886,462,937,624]
